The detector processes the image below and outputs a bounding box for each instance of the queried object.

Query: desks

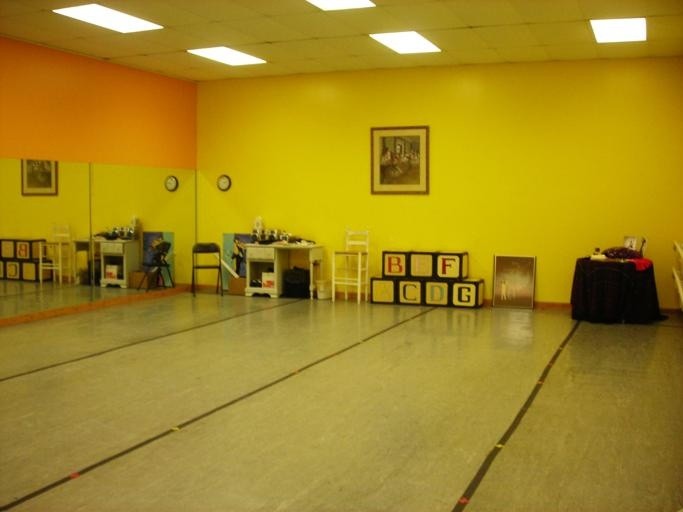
[243,243,322,299]
[569,257,661,326]
[71,237,141,289]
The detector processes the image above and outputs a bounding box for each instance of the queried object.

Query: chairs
[136,241,175,292]
[37,221,73,288]
[331,225,369,303]
[190,243,224,297]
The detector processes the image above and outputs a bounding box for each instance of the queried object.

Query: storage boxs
[262,272,274,289]
[229,277,247,295]
[106,264,122,279]
[130,271,150,289]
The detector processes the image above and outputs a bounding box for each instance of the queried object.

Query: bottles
[252,229,288,240]
[111,227,133,237]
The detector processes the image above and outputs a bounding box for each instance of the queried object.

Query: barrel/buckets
[315,279,332,300]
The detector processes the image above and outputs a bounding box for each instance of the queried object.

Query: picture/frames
[492,254,536,310]
[21,160,58,196]
[371,125,427,195]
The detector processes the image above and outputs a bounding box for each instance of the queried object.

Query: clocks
[216,174,231,192]
[164,176,178,191]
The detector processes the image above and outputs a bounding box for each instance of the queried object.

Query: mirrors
[0,158,197,319]
